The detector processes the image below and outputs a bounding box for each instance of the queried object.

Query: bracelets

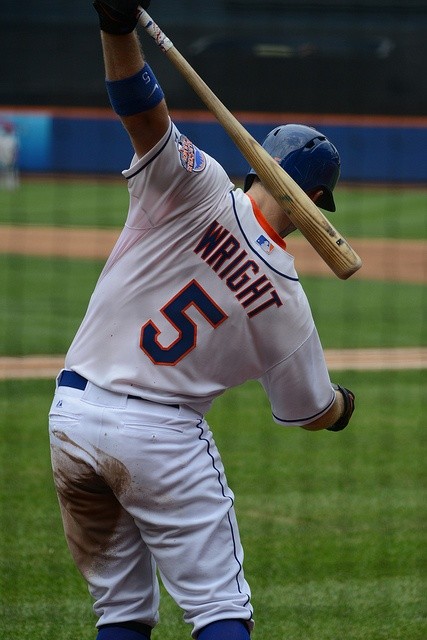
[104,60,165,118]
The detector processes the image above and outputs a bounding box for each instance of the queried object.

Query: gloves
[327,385,357,434]
[93,1,136,32]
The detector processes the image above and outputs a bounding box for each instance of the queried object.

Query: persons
[46,0,356,639]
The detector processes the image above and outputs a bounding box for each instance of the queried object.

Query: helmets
[246,119,342,214]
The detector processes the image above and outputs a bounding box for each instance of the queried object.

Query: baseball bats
[138,5,362,280]
